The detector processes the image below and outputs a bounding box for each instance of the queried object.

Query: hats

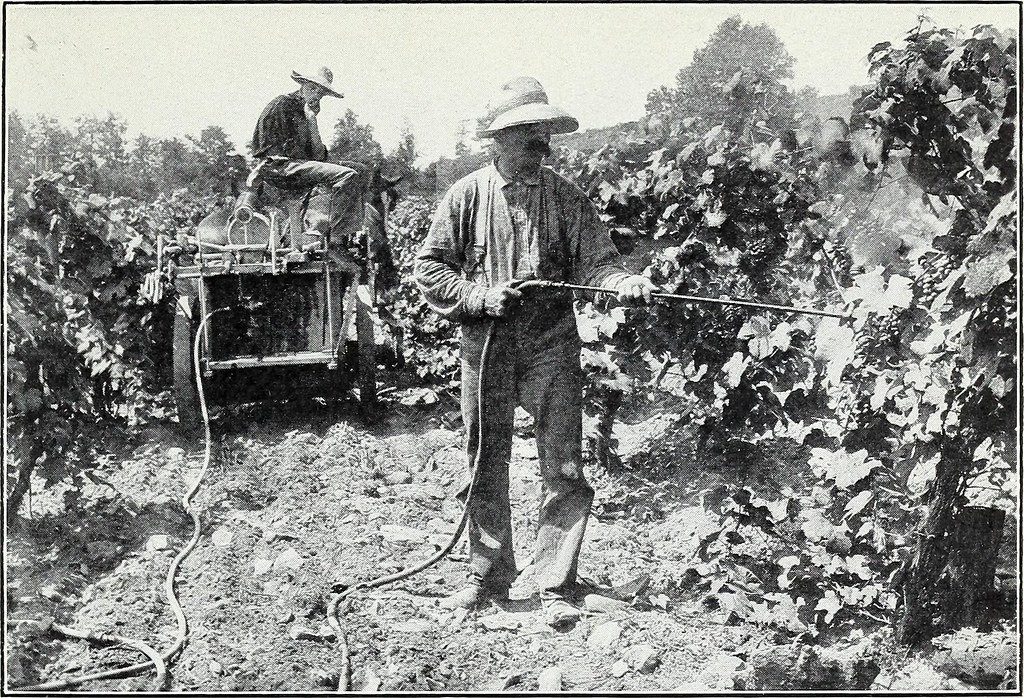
[289,64,344,103]
[477,74,579,141]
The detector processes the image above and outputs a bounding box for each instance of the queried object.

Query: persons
[410,72,660,625]
[250,64,371,273]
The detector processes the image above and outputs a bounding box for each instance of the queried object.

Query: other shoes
[438,578,509,609]
[327,240,353,269]
[540,595,577,627]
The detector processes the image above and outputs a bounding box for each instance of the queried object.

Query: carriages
[166,159,404,428]
